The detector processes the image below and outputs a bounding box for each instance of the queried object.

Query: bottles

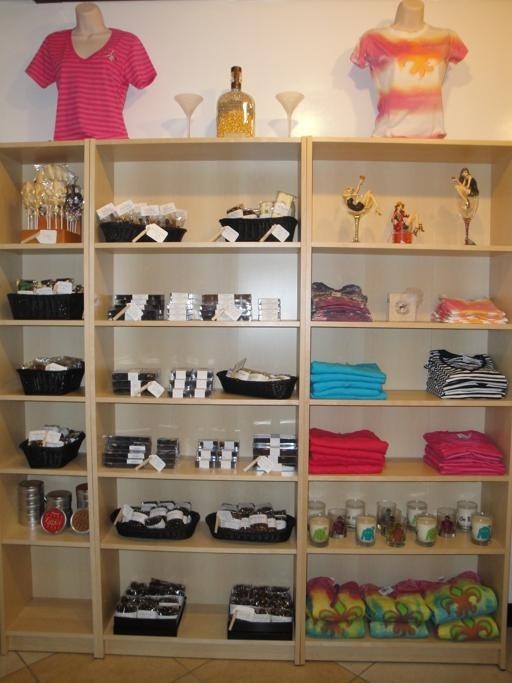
[216,66,255,137]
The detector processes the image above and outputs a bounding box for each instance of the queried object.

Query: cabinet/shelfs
[3,138,97,664]
[301,134,512,672]
[90,136,306,665]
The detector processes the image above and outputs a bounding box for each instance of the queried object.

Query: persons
[391,200,424,237]
[24,2,158,142]
[450,166,481,219]
[342,174,383,215]
[350,0,471,141]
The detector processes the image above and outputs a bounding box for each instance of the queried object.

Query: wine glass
[173,92,207,138]
[272,91,305,137]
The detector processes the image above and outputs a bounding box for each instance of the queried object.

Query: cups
[307,498,493,549]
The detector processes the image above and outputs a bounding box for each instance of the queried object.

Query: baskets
[99,221,186,241]
[109,505,200,539]
[216,369,298,398]
[18,430,87,469]
[15,367,84,395]
[7,293,84,320]
[220,217,299,242]
[206,510,297,542]
